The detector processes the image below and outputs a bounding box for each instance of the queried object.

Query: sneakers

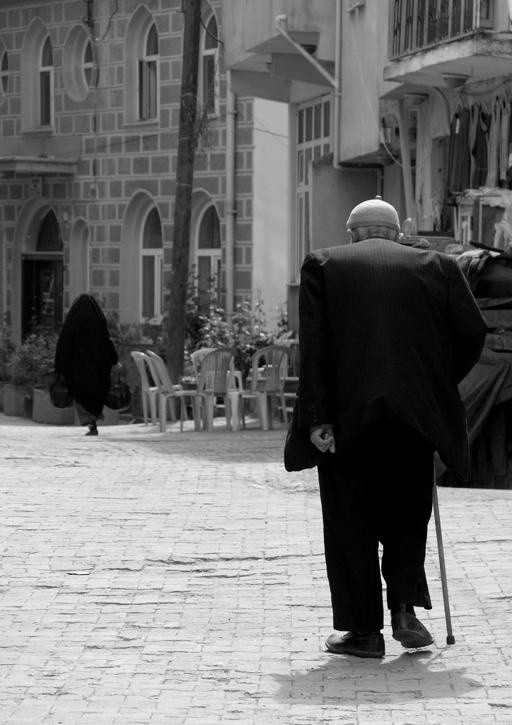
[325,630,385,657]
[86,428,98,435]
[391,613,433,648]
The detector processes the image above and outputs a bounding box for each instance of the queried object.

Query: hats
[347,196,401,229]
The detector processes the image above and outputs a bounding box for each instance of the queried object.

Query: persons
[283,197,486,655]
[55,293,117,438]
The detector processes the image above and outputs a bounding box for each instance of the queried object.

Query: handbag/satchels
[48,374,73,408]
[105,380,130,409]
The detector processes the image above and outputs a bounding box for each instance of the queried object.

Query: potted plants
[2,325,74,426]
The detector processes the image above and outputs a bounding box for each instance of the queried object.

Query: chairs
[129,340,299,431]
